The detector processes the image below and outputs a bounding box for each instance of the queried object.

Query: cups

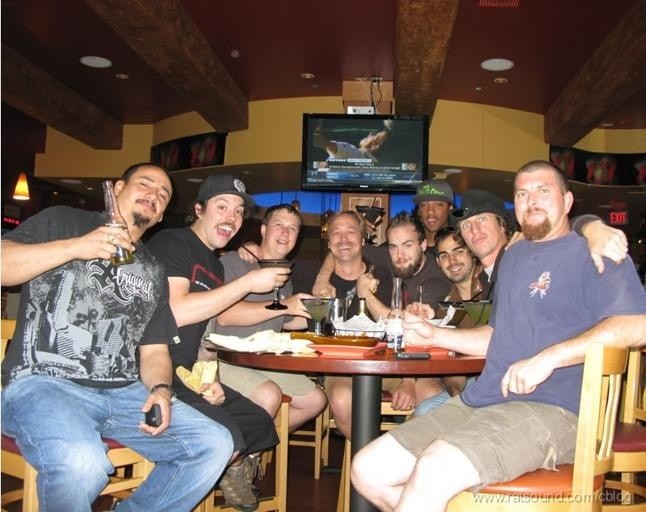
[299,297,334,336]
[256,258,294,311]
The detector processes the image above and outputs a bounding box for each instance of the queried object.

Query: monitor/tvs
[300,111,429,195]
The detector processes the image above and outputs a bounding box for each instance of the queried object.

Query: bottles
[358,298,366,315]
[386,278,405,356]
[102,181,134,266]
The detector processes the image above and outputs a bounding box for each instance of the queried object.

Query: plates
[290,332,379,347]
[306,343,389,360]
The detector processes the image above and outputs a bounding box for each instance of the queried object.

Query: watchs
[149,384,178,405]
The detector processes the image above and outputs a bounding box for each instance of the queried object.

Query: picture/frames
[339,192,390,249]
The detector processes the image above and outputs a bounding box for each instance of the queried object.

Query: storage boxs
[342,80,394,114]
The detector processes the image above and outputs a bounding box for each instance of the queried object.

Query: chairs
[321,378,416,470]
[615,338,646,506]
[0,316,161,512]
[175,394,292,511]
[448,342,627,512]
[295,389,322,480]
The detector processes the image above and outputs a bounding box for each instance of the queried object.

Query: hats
[412,179,454,204]
[450,190,510,233]
[196,172,256,207]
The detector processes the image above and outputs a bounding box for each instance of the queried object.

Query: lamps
[78,24,112,70]
[481,27,513,72]
[115,50,134,80]
[12,171,31,202]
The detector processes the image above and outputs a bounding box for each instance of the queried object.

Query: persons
[197,204,327,512]
[144,175,280,477]
[312,211,456,443]
[311,131,392,161]
[412,180,460,256]
[292,200,301,216]
[352,160,646,512]
[449,188,629,301]
[435,227,476,399]
[2,162,234,512]
[329,209,394,441]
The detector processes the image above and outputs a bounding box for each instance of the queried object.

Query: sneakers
[246,456,262,489]
[220,461,260,511]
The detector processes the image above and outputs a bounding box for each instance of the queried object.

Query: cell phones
[396,352,432,360]
[145,405,161,427]
